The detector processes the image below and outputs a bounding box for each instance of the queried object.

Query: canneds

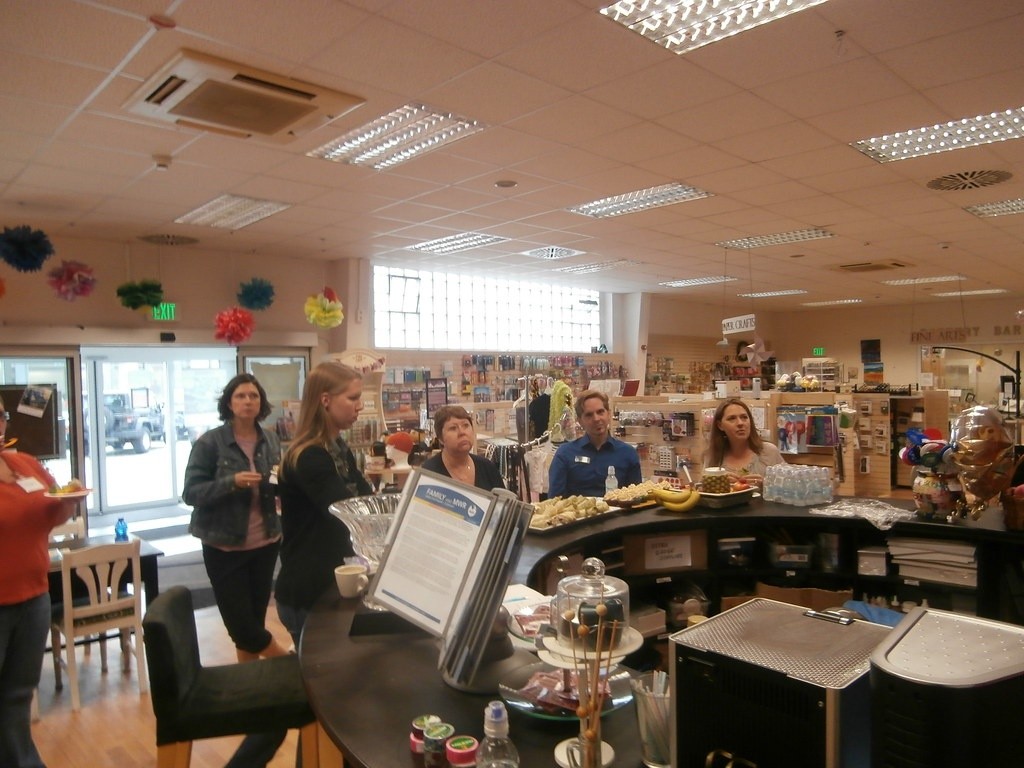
[409,714,480,768]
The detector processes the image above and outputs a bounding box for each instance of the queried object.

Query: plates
[43,489,91,498]
[497,667,634,720]
[506,600,550,644]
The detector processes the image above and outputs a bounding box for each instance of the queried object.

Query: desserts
[602,480,670,507]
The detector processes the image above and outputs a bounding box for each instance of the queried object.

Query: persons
[182,371,297,662]
[420,405,506,491]
[273,359,388,656]
[697,398,787,484]
[0,396,85,768]
[548,388,642,500]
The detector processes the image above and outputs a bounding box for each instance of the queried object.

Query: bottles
[763,462,832,507]
[605,465,618,493]
[474,700,521,768]
[445,734,479,768]
[409,714,442,768]
[114,519,128,543]
[422,722,456,768]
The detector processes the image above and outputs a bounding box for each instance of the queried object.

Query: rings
[246,481,250,487]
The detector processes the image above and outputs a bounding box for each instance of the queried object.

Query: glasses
[0,411,10,421]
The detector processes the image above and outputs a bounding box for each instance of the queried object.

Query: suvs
[81,391,163,455]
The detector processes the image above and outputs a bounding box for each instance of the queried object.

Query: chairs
[48,516,147,708]
[143,585,316,768]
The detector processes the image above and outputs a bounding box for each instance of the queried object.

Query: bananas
[651,481,700,512]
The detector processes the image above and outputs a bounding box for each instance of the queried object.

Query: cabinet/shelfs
[439,351,949,490]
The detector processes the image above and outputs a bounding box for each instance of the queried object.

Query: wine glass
[327,493,402,612]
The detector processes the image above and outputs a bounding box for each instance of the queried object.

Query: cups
[334,564,369,598]
[630,672,671,768]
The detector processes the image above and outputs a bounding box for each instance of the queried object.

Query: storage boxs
[770,537,815,569]
[632,605,667,640]
[622,532,707,574]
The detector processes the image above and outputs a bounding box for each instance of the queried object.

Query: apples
[732,481,751,491]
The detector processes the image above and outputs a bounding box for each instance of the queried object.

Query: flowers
[1,225,345,343]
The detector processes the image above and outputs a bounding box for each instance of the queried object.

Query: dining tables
[45,533,164,651]
[300,497,1005,768]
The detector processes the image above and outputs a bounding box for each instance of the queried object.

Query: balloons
[942,404,1024,521]
